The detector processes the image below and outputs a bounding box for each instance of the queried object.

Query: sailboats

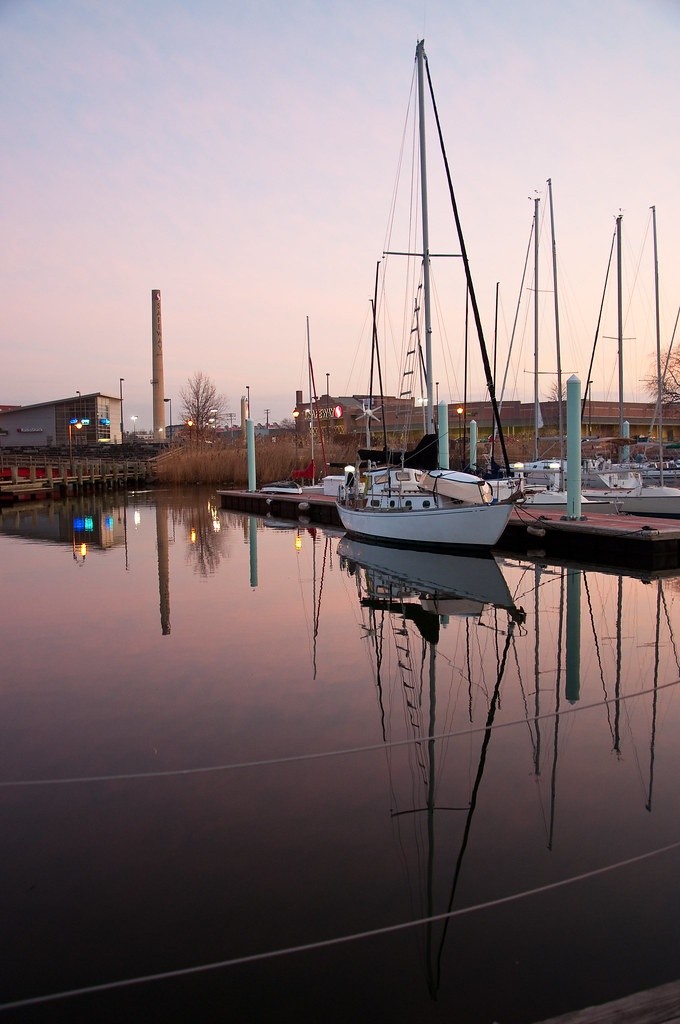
[215,178,680,543]
[335,532,528,1004]
[332,38,515,546]
